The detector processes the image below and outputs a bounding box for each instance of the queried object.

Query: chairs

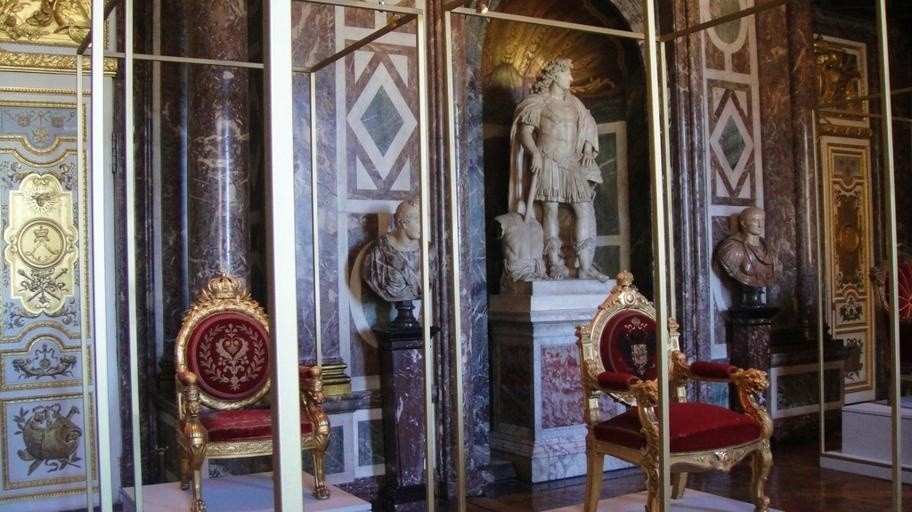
[173,270,334,511]
[572,269,781,511]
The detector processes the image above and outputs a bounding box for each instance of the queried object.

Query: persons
[363,199,433,304]
[715,206,774,287]
[493,58,609,283]
